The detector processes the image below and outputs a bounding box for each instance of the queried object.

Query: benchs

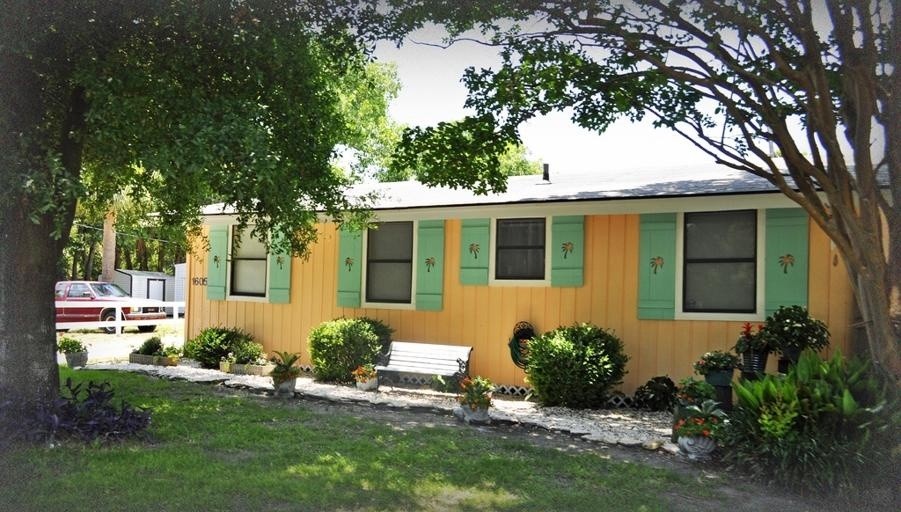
[373,341,473,394]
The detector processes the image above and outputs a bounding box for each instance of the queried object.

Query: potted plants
[760,304,831,373]
[57,336,89,368]
[130,337,183,367]
[218,341,276,378]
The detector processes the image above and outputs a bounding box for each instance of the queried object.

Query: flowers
[671,376,734,447]
[457,374,497,412]
[350,362,379,384]
[268,350,303,385]
[691,350,743,377]
[730,320,778,357]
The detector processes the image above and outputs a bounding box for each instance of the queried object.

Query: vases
[676,434,717,461]
[739,353,766,374]
[704,368,733,386]
[463,404,489,420]
[355,377,378,391]
[274,378,296,393]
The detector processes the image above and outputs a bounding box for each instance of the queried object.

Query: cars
[55,280,167,334]
